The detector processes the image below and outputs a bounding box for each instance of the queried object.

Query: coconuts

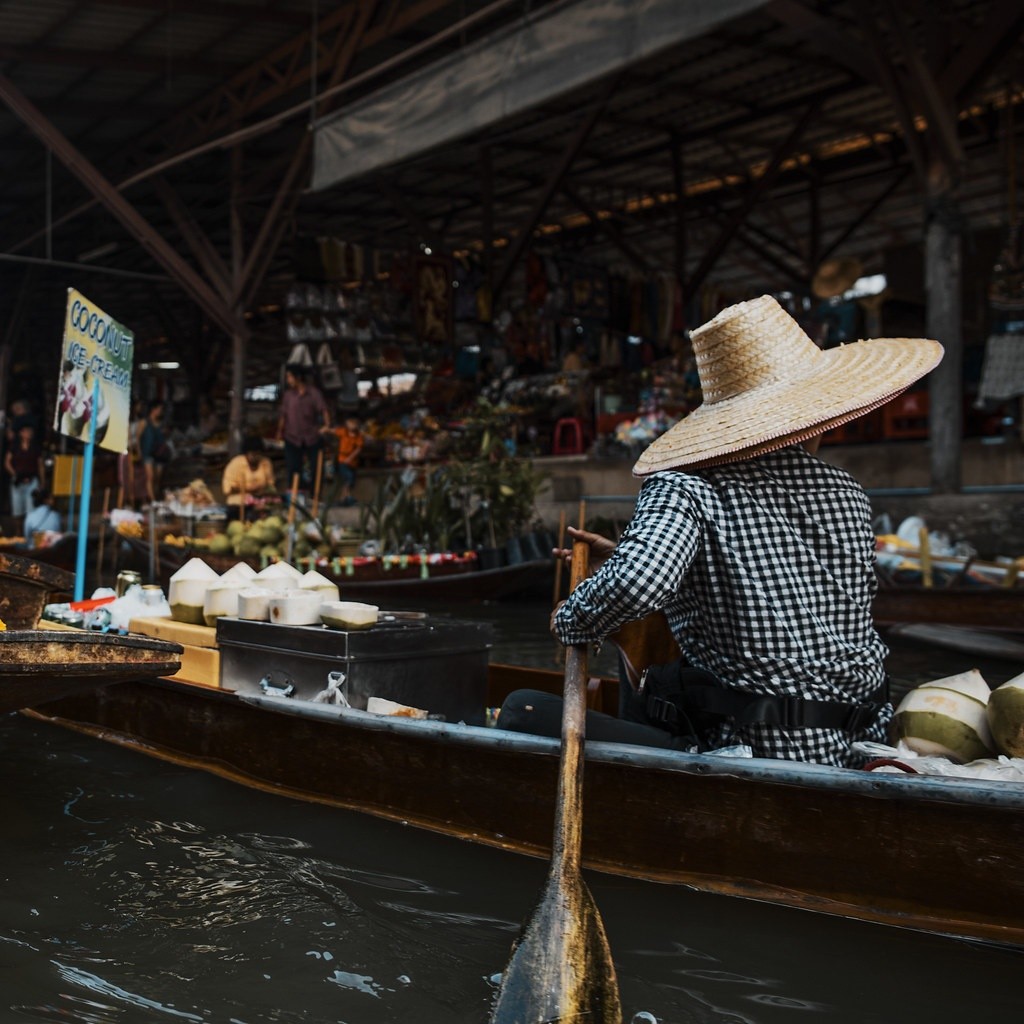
[886,668,1024,765]
[166,518,336,559]
[168,557,379,630]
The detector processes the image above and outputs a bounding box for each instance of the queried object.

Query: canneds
[115,570,141,598]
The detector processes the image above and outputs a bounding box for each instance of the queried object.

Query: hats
[630,294,946,475]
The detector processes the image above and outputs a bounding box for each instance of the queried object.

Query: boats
[0,472,1023,958]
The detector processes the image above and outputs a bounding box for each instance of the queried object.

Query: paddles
[491,542,622,1023]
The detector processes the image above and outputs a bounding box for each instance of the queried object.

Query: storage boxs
[217,612,493,728]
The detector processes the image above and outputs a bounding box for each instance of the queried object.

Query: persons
[326,419,363,507]
[116,399,168,509]
[274,365,330,500]
[17,487,62,552]
[494,295,944,773]
[0,403,46,517]
[221,432,274,497]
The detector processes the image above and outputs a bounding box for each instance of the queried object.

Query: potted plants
[359,434,558,571]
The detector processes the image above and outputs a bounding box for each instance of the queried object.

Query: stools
[555,418,592,455]
